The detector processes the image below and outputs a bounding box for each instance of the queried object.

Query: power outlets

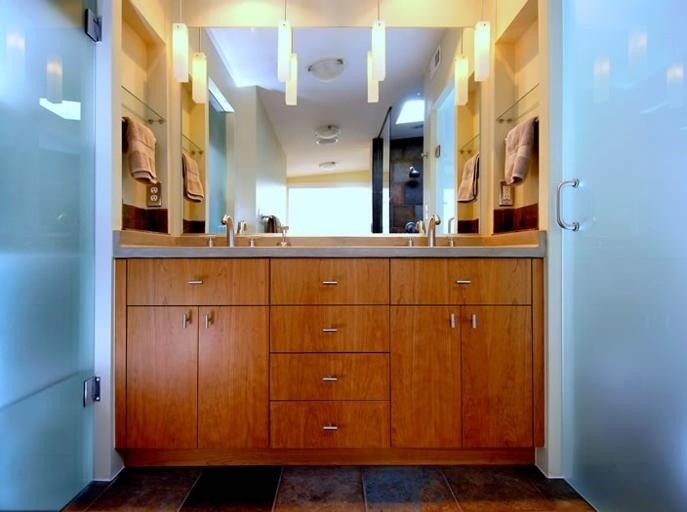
[145,182,162,207]
[498,180,515,206]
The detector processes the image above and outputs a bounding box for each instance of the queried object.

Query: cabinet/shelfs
[270,256,390,453]
[124,258,269,456]
[391,258,535,454]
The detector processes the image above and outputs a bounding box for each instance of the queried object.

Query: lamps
[314,122,340,171]
[367,26,379,103]
[306,57,345,80]
[191,27,209,105]
[276,0,293,83]
[453,24,468,107]
[284,52,299,105]
[190,23,470,109]
[472,2,492,81]
[368,1,387,81]
[409,151,429,177]
[169,2,190,82]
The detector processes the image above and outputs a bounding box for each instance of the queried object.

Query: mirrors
[176,21,484,237]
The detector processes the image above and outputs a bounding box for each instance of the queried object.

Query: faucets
[222,214,235,246]
[415,220,426,233]
[428,213,440,247]
[237,222,247,234]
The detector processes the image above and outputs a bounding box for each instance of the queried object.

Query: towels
[456,151,479,203]
[181,152,204,203]
[503,115,538,184]
[265,214,284,233]
[121,114,159,188]
